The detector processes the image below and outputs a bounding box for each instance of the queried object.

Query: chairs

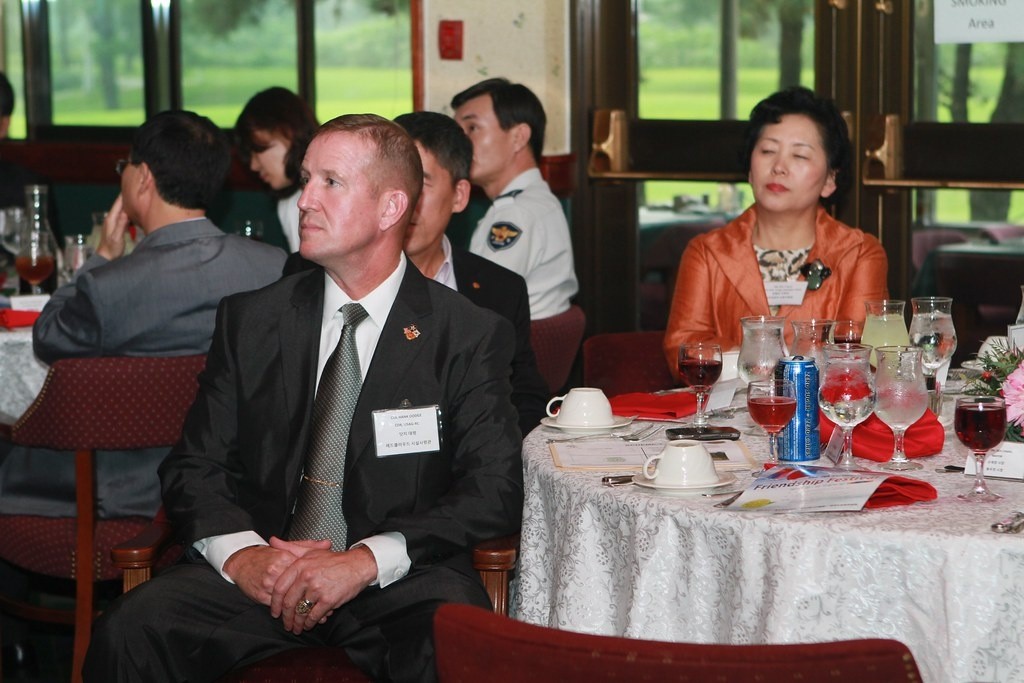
[0,305,922,683]
[909,224,1024,368]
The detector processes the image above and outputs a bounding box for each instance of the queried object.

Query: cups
[737,315,863,386]
[546,387,614,427]
[0,209,27,255]
[976,336,1008,367]
[860,299,909,368]
[63,233,93,283]
[721,351,740,381]
[644,440,719,487]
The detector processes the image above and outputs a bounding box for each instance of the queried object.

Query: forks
[548,424,665,443]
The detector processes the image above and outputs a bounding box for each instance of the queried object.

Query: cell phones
[666,426,741,441]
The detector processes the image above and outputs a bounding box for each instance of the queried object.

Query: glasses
[115,159,142,175]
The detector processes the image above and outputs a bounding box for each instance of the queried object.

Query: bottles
[19,184,59,293]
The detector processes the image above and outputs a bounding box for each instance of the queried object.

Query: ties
[288,303,370,551]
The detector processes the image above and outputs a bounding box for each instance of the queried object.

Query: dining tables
[507,380,1024,683]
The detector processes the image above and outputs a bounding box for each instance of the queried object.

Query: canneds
[768,356,821,463]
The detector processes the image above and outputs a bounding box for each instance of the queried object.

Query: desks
[0,323,52,421]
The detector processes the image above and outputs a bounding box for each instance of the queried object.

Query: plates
[632,470,735,495]
[540,415,632,434]
[961,360,986,370]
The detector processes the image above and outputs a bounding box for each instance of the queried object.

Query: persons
[450,77,580,319]
[0,109,297,517]
[0,70,63,293]
[236,85,319,259]
[395,111,552,430]
[81,114,523,683]
[664,86,891,388]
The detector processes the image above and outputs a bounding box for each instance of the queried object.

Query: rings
[302,596,314,607]
[297,601,311,615]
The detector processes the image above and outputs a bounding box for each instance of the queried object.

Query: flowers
[952,338,1023,446]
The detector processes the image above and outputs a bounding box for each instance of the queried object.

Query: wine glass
[677,343,722,428]
[954,397,1006,503]
[873,346,929,470]
[16,231,54,294]
[747,379,797,470]
[817,343,876,473]
[909,297,958,392]
